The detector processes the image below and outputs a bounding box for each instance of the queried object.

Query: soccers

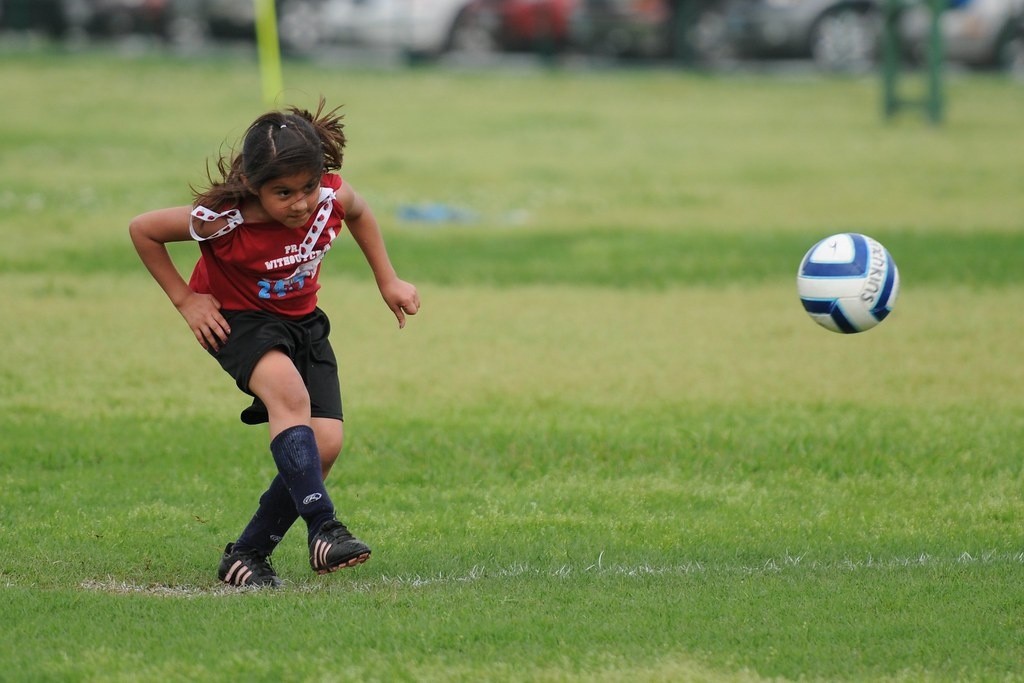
[794,231,903,336]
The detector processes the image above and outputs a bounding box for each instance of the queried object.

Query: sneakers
[219,541,281,590]
[308,519,370,577]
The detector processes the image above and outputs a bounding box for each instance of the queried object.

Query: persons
[493,0,728,75]
[129,95,421,587]
